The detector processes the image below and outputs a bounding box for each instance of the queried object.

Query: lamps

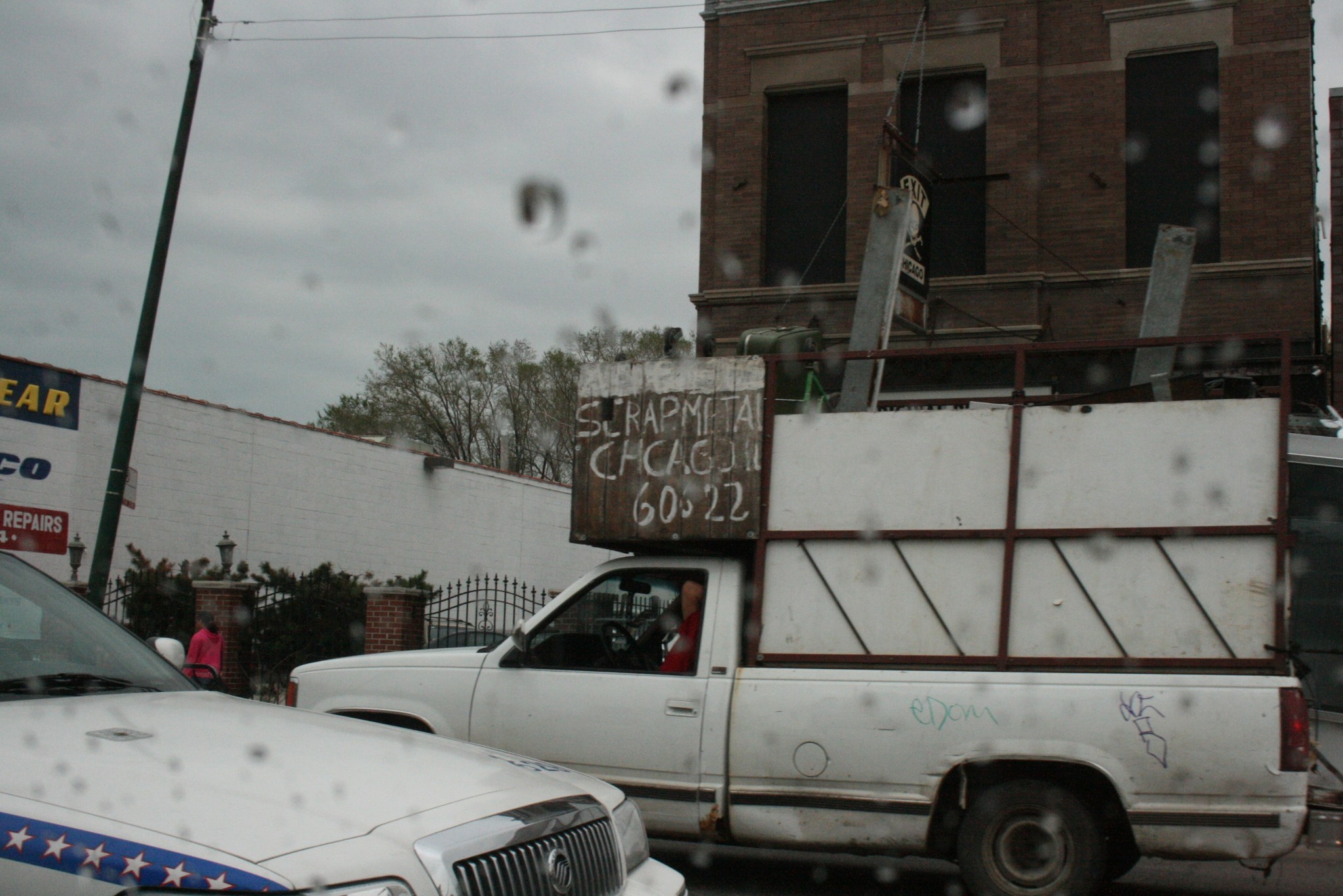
[67,533,87,580]
[215,529,237,573]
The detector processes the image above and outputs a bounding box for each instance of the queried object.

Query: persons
[182,610,225,690]
[661,581,703,671]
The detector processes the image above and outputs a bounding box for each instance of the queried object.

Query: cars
[0,549,691,896]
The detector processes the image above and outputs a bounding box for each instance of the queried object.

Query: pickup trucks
[285,327,1311,896]
